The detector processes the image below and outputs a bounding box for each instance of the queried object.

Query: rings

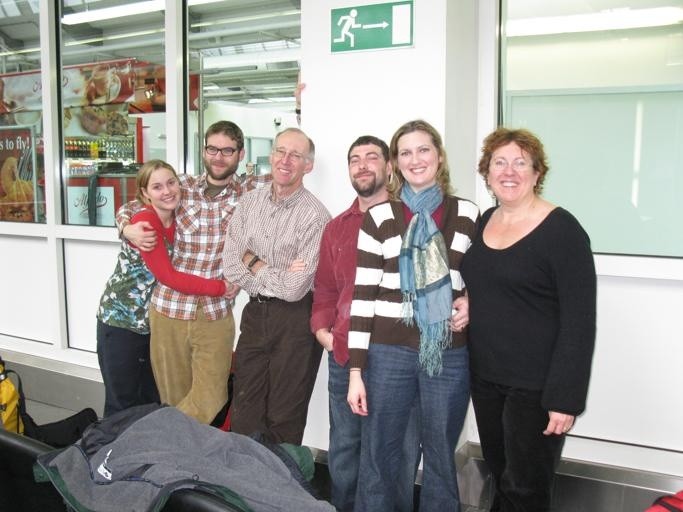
[347,120,481,511]
[461,325,464,331]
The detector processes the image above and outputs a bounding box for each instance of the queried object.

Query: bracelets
[247,256,259,269]
[349,369,361,372]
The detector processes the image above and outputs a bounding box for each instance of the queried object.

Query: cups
[91,64,116,97]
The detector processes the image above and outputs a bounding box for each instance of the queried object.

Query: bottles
[65,162,94,176]
[65,137,132,159]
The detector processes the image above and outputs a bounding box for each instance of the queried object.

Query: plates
[82,74,121,104]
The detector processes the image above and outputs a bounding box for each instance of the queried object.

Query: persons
[241,163,254,177]
[295,83,305,125]
[459,130,596,512]
[115,121,273,426]
[310,136,420,512]
[96,159,238,419]
[222,128,333,445]
